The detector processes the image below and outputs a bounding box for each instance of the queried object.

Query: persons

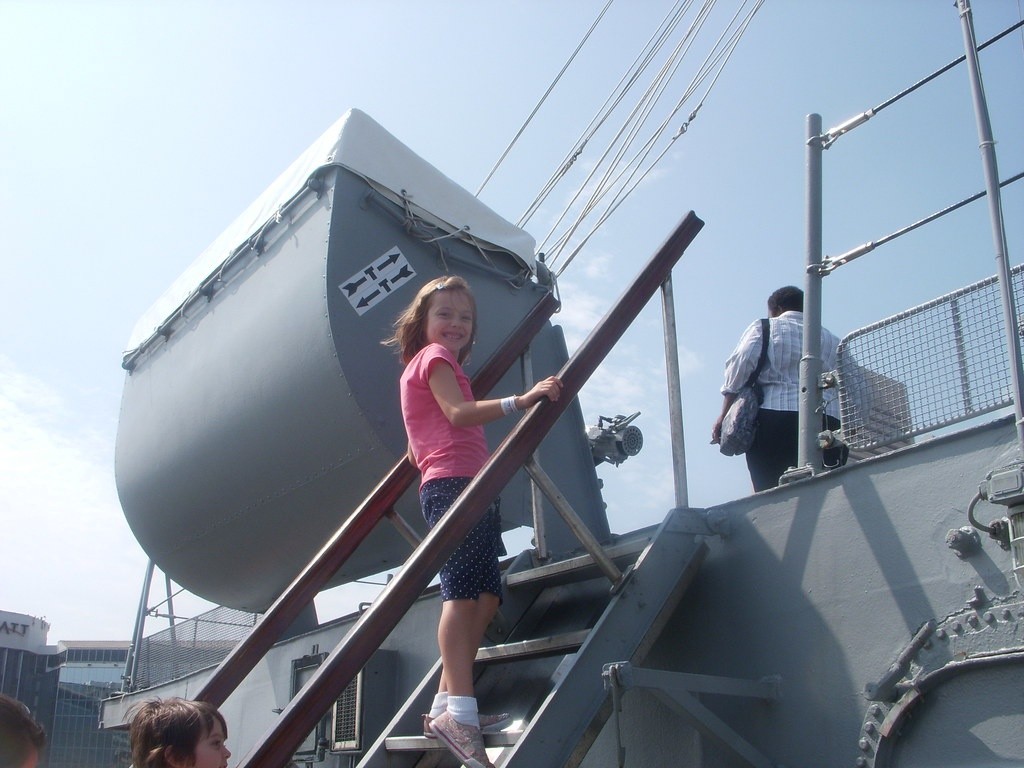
[124,697,231,768]
[0,693,47,768]
[711,286,841,493]
[380,276,563,768]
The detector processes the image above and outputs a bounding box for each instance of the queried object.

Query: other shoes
[421,713,513,738]
[428,708,494,768]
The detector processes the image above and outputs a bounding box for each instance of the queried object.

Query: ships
[97,0,1024,768]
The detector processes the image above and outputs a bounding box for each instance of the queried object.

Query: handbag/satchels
[720,319,770,457]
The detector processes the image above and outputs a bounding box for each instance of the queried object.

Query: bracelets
[501,395,519,416]
